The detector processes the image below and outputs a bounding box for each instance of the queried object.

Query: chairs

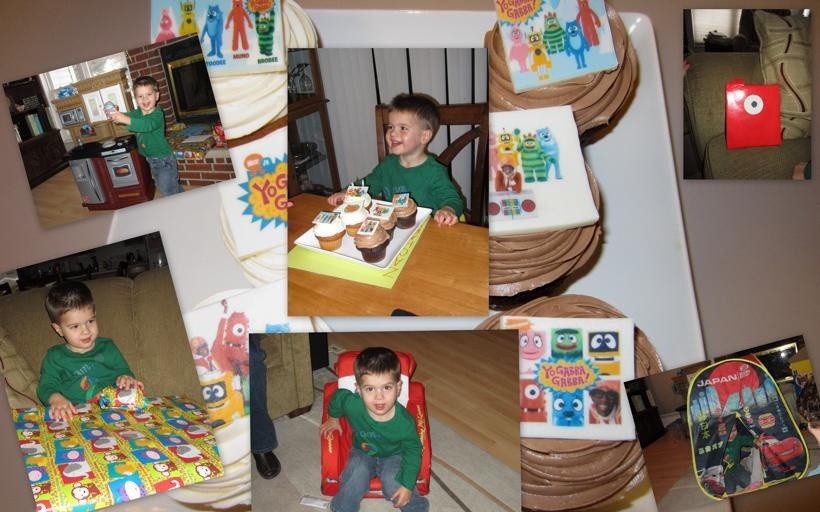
[375,103,489,225]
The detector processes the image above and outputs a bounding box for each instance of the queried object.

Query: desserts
[148,0,322,512]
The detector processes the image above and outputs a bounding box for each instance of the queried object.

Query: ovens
[103,152,139,189]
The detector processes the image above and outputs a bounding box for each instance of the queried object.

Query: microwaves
[58,107,86,127]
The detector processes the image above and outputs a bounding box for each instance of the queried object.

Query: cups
[104,105,115,120]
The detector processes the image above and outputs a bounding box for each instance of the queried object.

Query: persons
[722,424,759,494]
[37,278,144,423]
[249,333,282,479]
[795,375,820,446]
[104,76,184,198]
[325,91,467,227]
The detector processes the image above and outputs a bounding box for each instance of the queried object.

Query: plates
[294,194,433,269]
[291,141,318,157]
[82,14,731,509]
[102,141,116,148]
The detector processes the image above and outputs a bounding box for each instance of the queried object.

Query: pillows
[753,10,812,138]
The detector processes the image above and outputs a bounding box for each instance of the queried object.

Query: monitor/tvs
[166,52,218,120]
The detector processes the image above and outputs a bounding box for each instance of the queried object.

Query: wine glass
[289,62,313,99]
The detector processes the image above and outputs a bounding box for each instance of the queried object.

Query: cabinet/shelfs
[3,75,72,192]
[288,48,340,194]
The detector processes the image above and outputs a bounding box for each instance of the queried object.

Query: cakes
[489,104,602,311]
[483,0,639,145]
[476,293,667,511]
[311,185,418,263]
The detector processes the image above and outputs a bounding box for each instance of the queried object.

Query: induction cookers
[100,134,136,156]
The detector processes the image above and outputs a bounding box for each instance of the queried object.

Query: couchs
[1,267,206,512]
